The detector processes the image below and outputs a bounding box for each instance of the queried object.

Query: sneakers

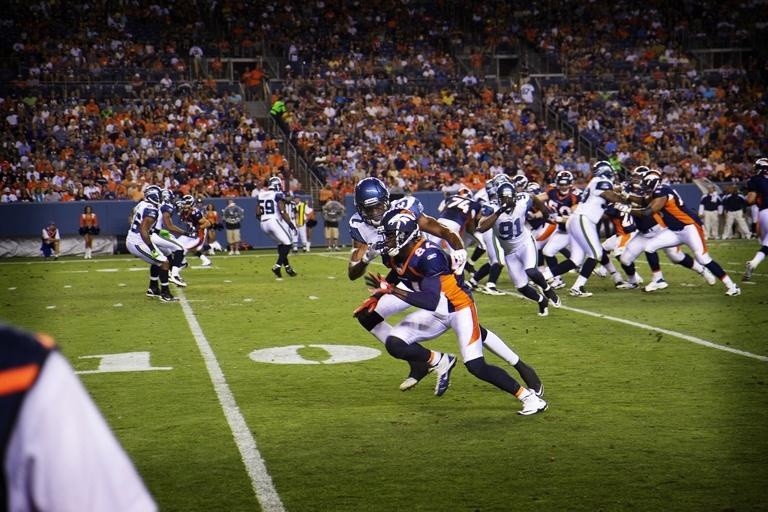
[568,286,593,298]
[272,264,295,277]
[517,366,548,415]
[529,267,566,316]
[742,260,754,280]
[464,261,504,296]
[592,266,606,278]
[615,274,668,292]
[147,261,188,301]
[701,265,717,284]
[400,353,457,396]
[725,282,741,296]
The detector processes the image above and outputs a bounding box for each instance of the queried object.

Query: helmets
[632,165,662,196]
[754,158,768,175]
[144,185,194,212]
[592,161,614,179]
[457,188,473,198]
[485,175,541,212]
[268,177,282,190]
[354,177,421,256]
[556,171,573,194]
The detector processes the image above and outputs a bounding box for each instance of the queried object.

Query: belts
[642,228,653,234]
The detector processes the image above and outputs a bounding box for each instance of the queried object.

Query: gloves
[452,249,467,274]
[183,226,199,238]
[160,230,169,237]
[614,203,632,213]
[152,249,159,259]
[365,273,394,295]
[353,296,378,317]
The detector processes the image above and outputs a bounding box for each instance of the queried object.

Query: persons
[349,178,544,396]
[353,207,548,415]
[2,324,162,511]
[0,0,768,317]
